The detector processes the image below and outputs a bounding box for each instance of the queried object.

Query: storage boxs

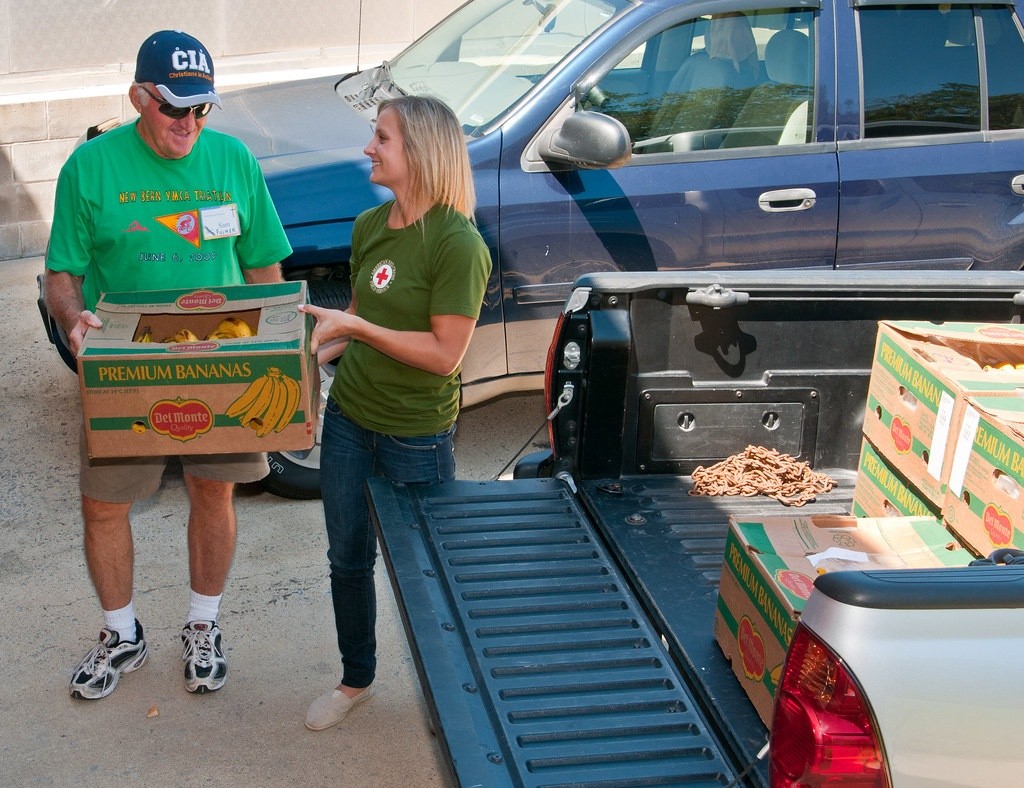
[943,395,1024,566]
[862,320,1024,511]
[711,511,975,730]
[75,281,321,461]
[849,433,944,518]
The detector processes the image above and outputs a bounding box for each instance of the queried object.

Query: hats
[135,29,223,112]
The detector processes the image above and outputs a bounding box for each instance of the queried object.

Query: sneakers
[180,618,227,694]
[67,618,149,700]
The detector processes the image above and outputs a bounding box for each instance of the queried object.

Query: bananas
[134,317,257,343]
[981,360,1024,371]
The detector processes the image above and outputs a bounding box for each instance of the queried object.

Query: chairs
[718,30,808,149]
[642,10,760,152]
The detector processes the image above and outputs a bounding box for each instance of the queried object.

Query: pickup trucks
[354,264,1024,786]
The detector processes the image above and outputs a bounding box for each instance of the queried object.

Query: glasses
[140,85,213,120]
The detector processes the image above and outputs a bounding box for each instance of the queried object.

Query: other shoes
[304,678,375,730]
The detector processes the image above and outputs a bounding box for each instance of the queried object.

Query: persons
[304,96,492,731]
[44,30,295,701]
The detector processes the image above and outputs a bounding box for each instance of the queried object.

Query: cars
[35,2,1024,503]
